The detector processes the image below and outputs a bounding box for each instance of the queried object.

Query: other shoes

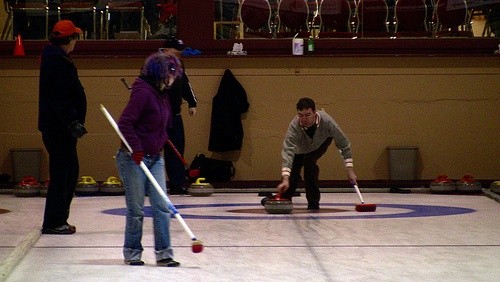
[42,222,76,234]
[157,257,180,267]
[306,202,320,213]
[123,259,144,265]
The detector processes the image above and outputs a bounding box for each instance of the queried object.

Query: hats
[163,36,187,50]
[51,20,82,38]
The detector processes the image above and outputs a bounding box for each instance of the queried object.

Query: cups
[292,38,304,55]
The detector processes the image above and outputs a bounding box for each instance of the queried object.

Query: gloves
[71,122,88,138]
[131,151,144,166]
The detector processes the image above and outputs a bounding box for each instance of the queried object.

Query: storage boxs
[213,21,244,40]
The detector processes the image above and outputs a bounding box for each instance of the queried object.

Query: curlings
[264,194,293,214]
[430,174,456,194]
[39,177,52,196]
[457,174,483,194]
[74,176,101,195]
[100,176,125,194]
[14,175,39,195]
[187,178,215,196]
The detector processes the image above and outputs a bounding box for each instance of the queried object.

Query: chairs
[10,1,49,39]
[58,0,96,41]
[105,2,146,40]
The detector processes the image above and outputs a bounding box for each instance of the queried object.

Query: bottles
[307,36,315,53]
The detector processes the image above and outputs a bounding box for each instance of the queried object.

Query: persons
[278,97,358,211]
[157,37,200,195]
[37,20,88,234]
[115,52,183,267]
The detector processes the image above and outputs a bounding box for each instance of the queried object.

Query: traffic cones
[12,34,25,57]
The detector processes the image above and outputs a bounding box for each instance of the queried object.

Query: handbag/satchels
[185,153,236,184]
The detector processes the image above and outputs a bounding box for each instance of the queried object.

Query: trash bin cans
[9,147,41,183]
[386,146,418,180]
